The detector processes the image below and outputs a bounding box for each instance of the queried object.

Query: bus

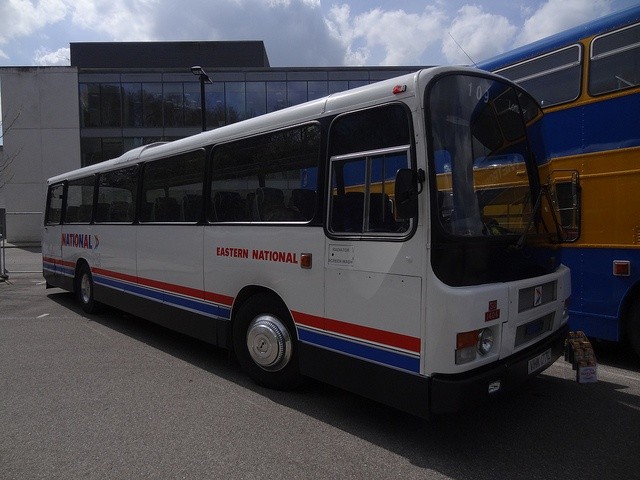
[41,65,571,419]
[298,4,639,369]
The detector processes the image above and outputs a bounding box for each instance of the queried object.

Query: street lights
[190,65,212,132]
